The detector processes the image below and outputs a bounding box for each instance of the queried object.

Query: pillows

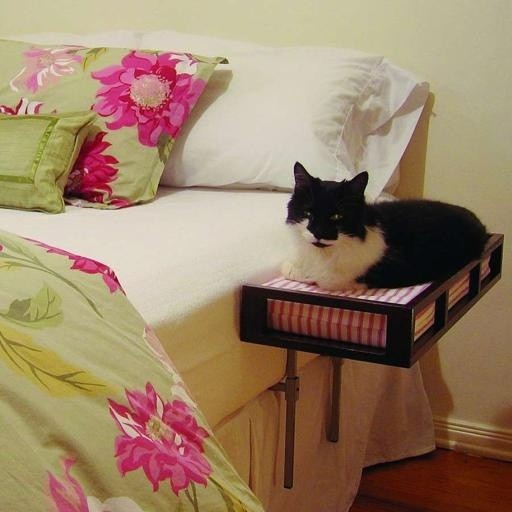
[137,38,430,205]
[1,38,229,211]
[1,110,96,214]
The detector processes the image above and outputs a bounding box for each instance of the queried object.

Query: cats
[279,161,491,291]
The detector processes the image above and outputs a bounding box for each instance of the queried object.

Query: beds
[0,185,440,512]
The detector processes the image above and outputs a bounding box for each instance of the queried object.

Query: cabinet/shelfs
[239,234,504,488]
